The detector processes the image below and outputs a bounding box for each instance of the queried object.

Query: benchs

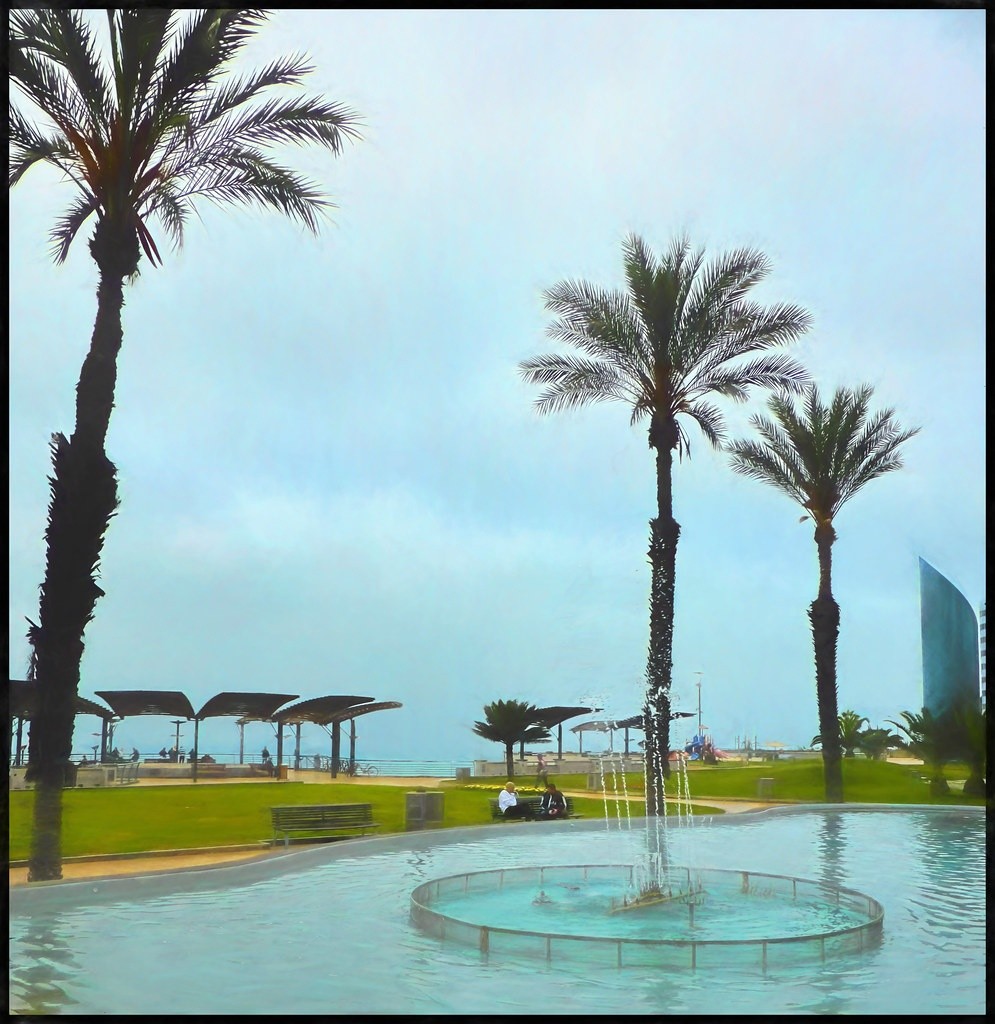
[191,764,228,778]
[488,797,584,823]
[268,804,382,851]
[248,763,270,778]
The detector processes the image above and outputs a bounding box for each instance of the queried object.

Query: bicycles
[323,757,349,773]
[354,762,379,777]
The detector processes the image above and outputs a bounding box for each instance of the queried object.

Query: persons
[534,754,549,790]
[80,747,194,765]
[261,747,274,777]
[499,782,538,821]
[539,784,569,820]
